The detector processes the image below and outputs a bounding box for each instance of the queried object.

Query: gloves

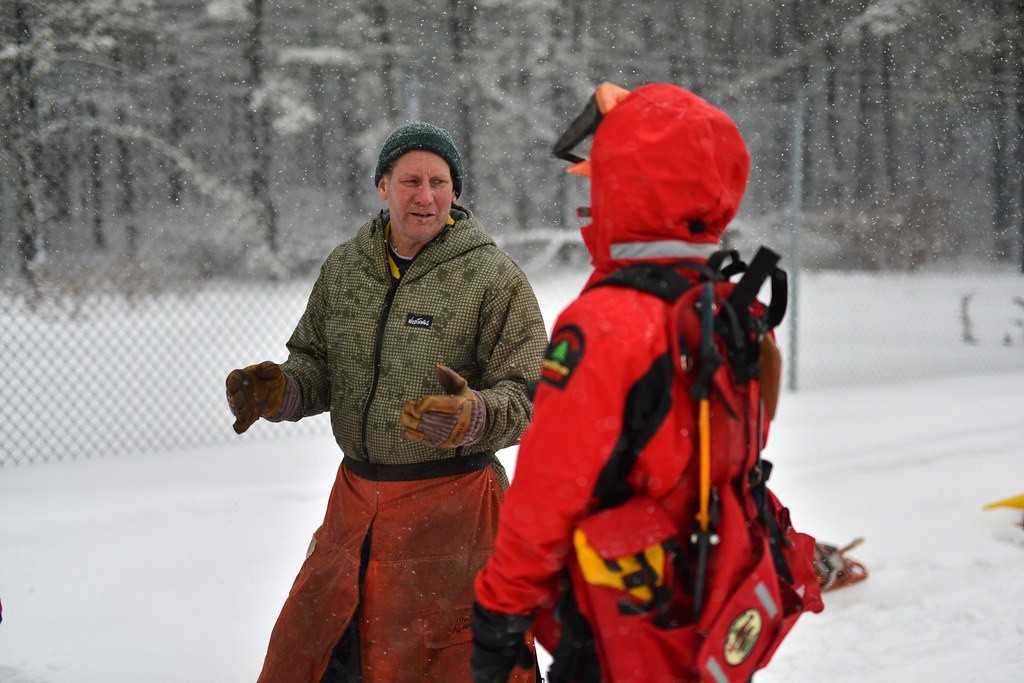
[400,365,488,449]
[471,600,541,683]
[226,361,300,434]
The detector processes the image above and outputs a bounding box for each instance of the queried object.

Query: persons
[226,122,548,682]
[470,83,823,683]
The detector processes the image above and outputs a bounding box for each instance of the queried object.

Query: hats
[550,81,631,177]
[374,122,463,200]
